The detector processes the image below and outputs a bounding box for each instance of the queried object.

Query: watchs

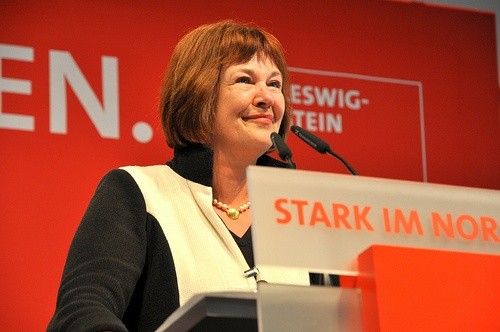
[43,19,341,332]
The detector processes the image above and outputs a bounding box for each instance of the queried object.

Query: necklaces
[212,195,251,220]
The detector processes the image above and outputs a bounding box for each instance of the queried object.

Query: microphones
[291,125,358,175]
[271,132,295,169]
